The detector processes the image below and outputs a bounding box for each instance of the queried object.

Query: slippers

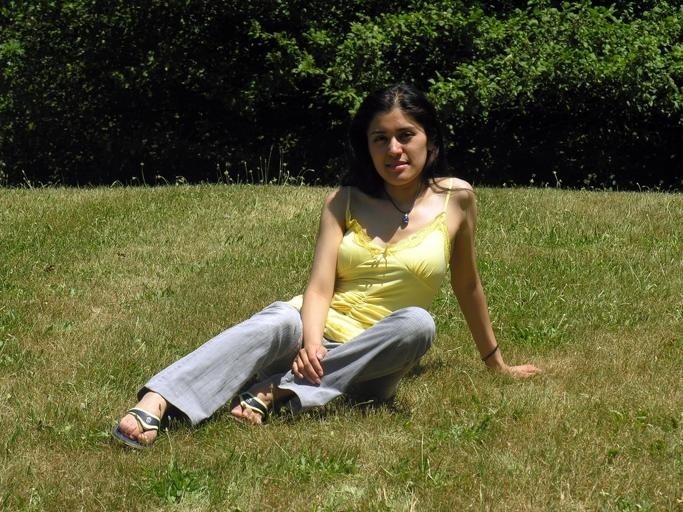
[111,405,162,451]
[227,387,269,427]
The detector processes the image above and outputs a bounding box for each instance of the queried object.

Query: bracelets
[482,344,499,363]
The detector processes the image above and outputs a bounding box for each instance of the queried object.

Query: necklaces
[381,182,424,226]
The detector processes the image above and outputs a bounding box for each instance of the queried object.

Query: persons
[112,84,541,449]
